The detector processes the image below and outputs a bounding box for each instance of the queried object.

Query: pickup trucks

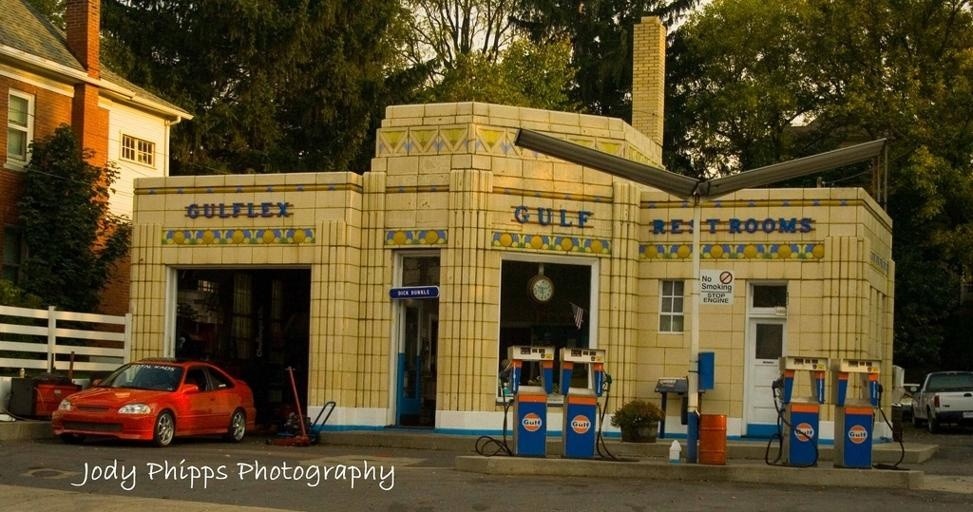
[910,370,972,435]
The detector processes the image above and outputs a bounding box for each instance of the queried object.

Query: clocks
[526,275,555,304]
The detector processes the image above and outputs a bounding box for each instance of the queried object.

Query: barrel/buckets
[699,414,726,465]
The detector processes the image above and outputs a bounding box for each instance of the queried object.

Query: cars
[51,356,256,448]
[899,382,921,412]
